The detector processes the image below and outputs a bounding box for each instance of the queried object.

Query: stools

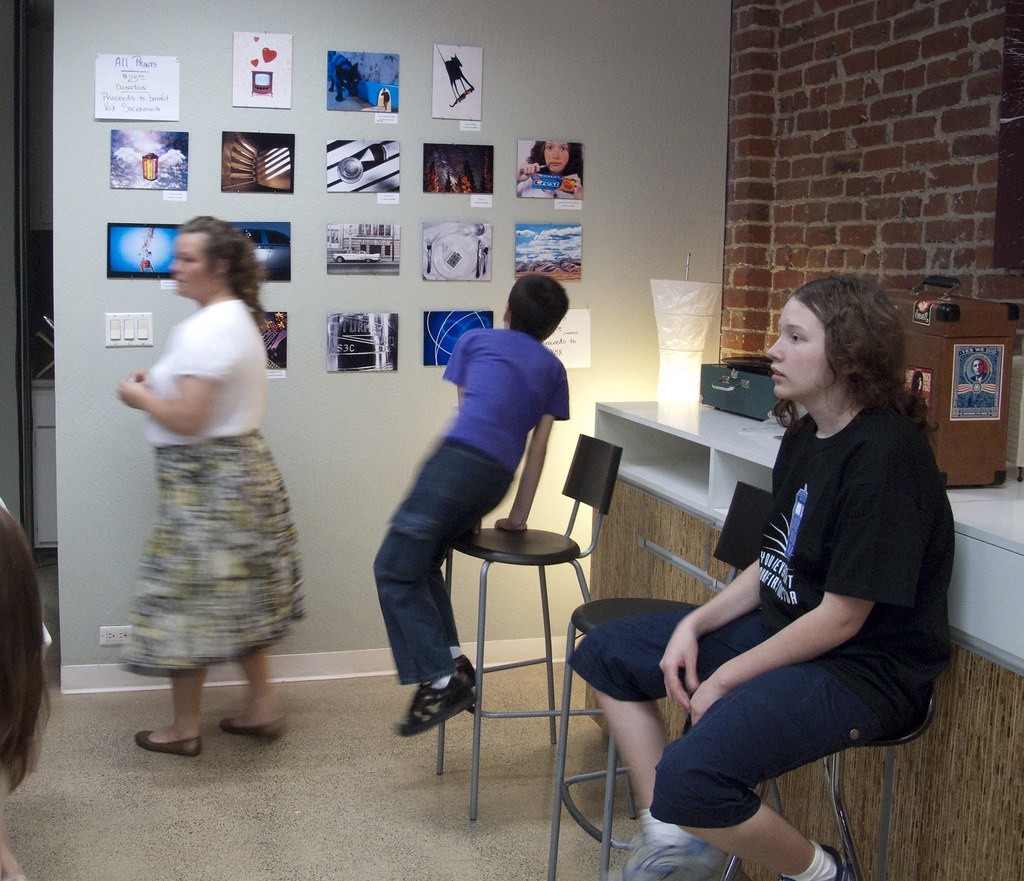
[435,433,625,823]
[545,481,933,881]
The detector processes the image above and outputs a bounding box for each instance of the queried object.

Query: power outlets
[100,624,134,644]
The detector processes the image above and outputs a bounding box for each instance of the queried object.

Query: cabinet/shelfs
[586,400,1024,880]
[31,378,59,548]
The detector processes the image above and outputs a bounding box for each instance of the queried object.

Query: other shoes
[453,655,476,688]
[218,714,288,739]
[400,677,475,736]
[779,844,855,881]
[135,730,202,756]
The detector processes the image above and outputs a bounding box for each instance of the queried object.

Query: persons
[516,140,583,199]
[569,275,956,881]
[0,502,43,881]
[371,274,569,736]
[118,215,286,756]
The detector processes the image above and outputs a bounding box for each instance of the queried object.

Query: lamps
[648,252,723,404]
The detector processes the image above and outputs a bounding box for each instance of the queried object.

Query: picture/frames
[107,222,185,280]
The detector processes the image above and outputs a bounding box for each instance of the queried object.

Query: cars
[240,230,291,276]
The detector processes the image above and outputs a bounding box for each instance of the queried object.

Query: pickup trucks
[332,248,381,263]
